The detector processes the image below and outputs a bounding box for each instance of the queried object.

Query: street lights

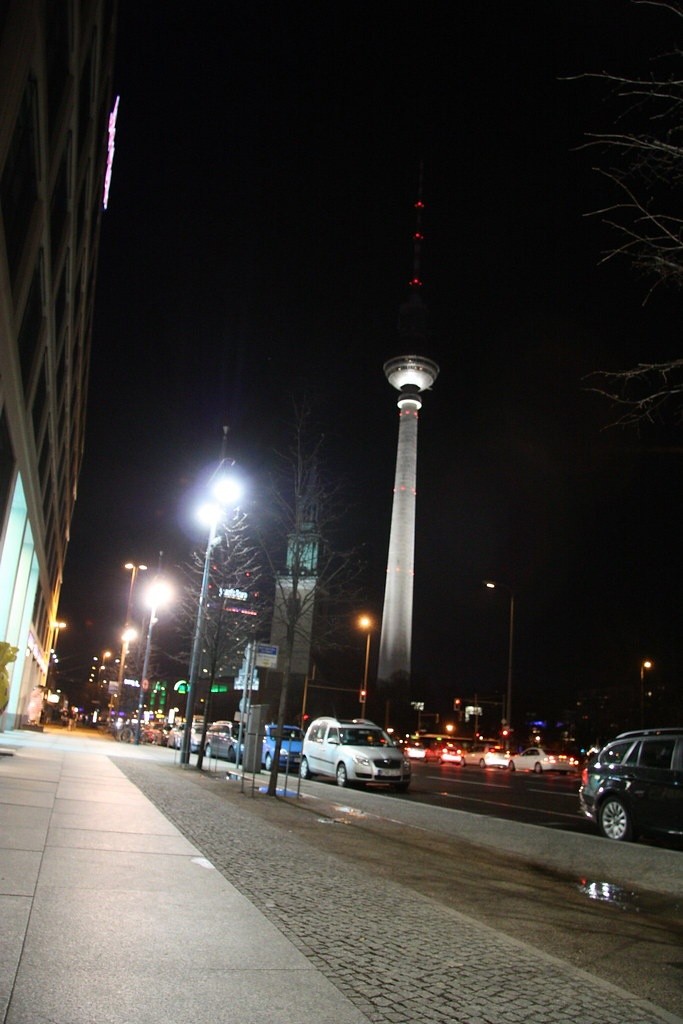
[178,475,243,764]
[109,562,149,735]
[358,614,373,719]
[639,660,652,729]
[484,579,515,737]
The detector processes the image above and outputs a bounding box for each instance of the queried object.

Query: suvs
[576,726,683,849]
[203,719,248,764]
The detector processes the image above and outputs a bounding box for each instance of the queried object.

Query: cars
[424,736,469,765]
[403,739,426,761]
[260,723,305,772]
[298,715,412,794]
[160,722,205,754]
[459,737,512,769]
[508,746,579,776]
